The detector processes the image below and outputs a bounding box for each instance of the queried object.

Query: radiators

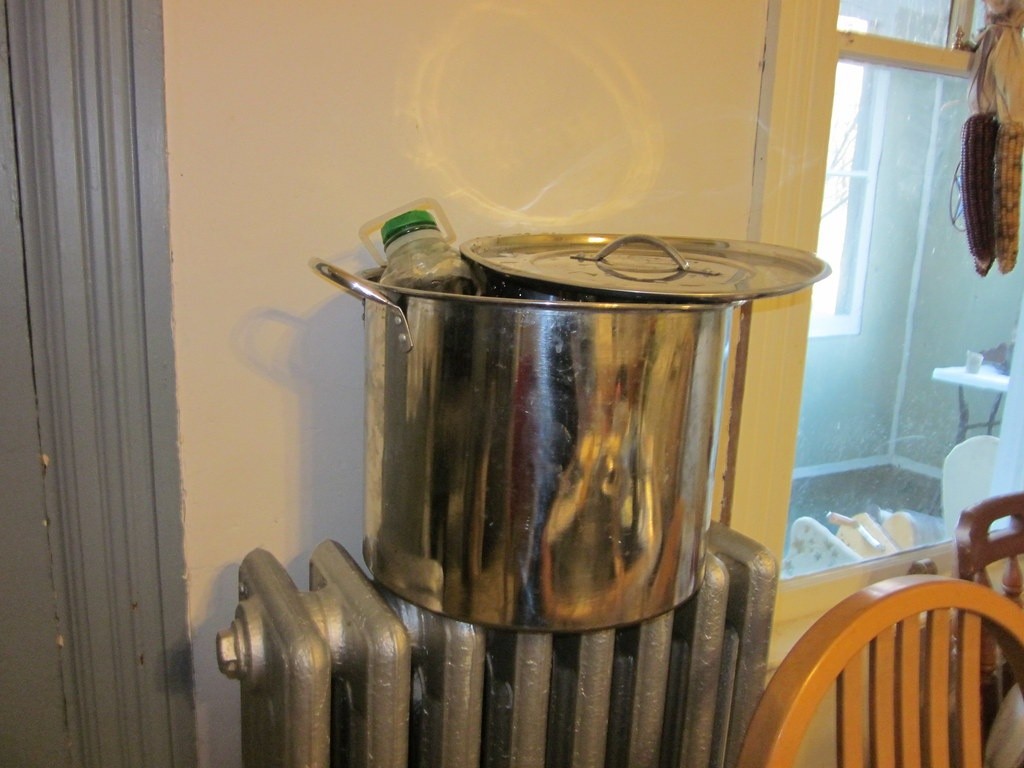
[215,519,778,768]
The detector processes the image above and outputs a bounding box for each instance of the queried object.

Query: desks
[932,366,1011,447]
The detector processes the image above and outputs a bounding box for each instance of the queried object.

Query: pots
[307,230,835,635]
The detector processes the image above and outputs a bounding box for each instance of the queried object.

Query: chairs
[733,573,1024,768]
[952,489,1024,747]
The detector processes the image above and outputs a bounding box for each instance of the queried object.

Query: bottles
[358,194,528,302]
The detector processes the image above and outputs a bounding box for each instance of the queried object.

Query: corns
[959,113,1024,276]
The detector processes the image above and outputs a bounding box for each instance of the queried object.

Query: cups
[964,352,984,374]
